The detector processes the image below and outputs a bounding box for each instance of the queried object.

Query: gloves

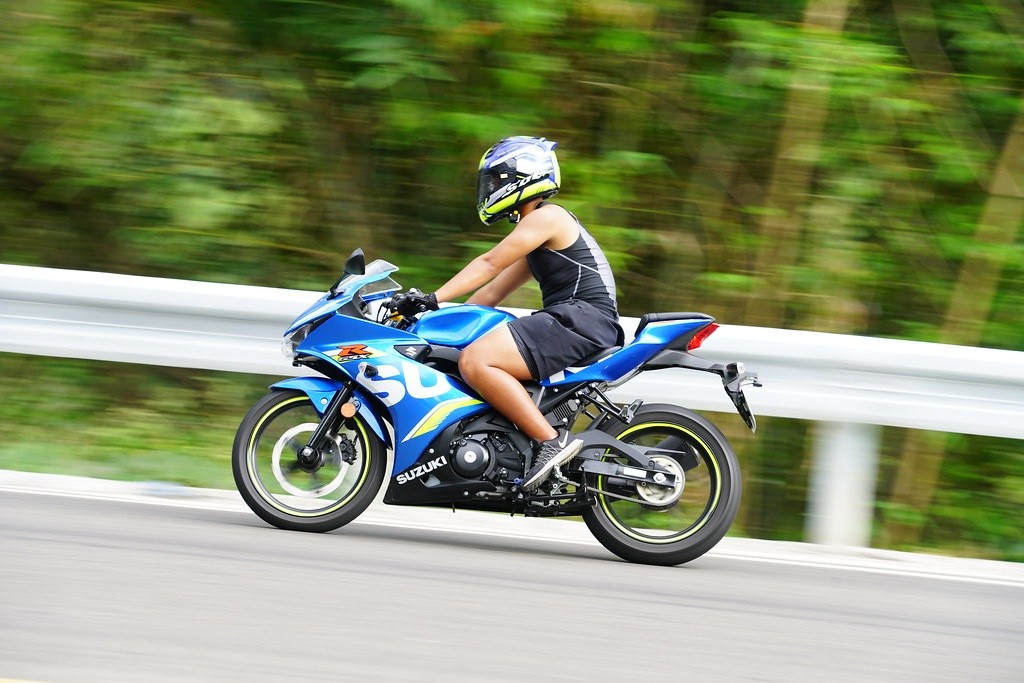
[395,288,440,318]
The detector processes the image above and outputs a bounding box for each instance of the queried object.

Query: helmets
[477,136,561,226]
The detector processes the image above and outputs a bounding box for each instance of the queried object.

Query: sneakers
[521,428,584,491]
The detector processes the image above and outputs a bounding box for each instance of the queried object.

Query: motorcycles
[230,243,765,566]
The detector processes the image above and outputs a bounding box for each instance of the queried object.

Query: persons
[392,137,617,490]
